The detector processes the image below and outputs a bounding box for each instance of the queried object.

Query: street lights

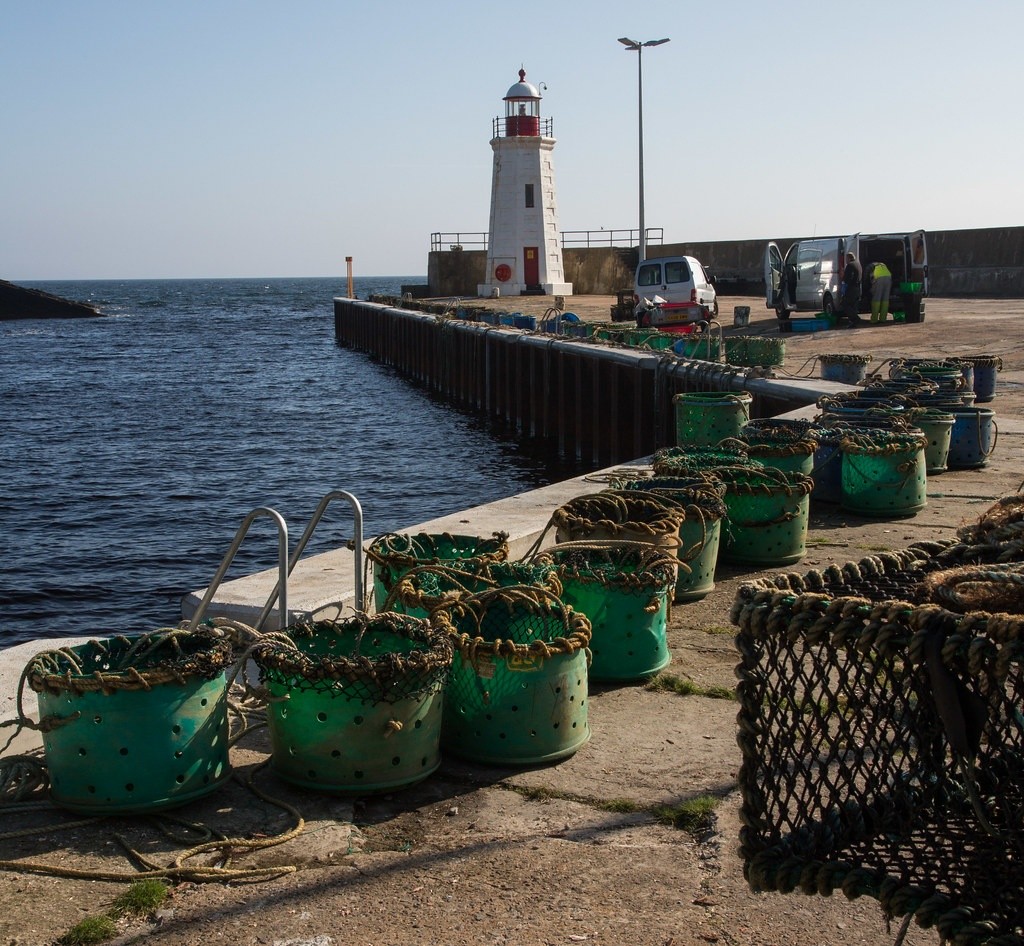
[616,36,672,269]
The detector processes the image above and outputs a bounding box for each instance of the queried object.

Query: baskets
[793,312,836,331]
[893,280,925,322]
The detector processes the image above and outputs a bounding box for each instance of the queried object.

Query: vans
[763,230,930,321]
[634,255,721,319]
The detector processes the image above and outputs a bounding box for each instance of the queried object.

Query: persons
[840,252,862,325]
[863,262,891,324]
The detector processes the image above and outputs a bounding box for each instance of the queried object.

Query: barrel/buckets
[733,306,751,326]
[554,296,565,311]
[491,287,500,298]
